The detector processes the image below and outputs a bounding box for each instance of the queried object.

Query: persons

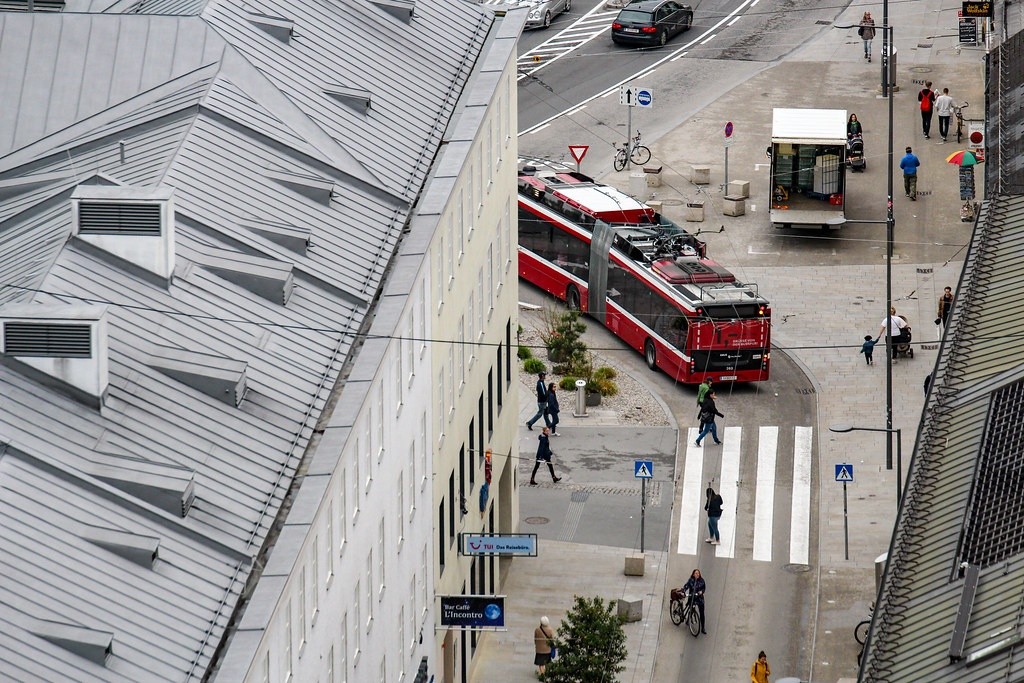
[900,147,920,200]
[525,371,557,430]
[860,334,880,365]
[681,568,707,634]
[530,428,562,485]
[937,286,955,327]
[533,616,555,675]
[859,11,876,62]
[704,488,723,544]
[696,376,717,434]
[847,113,862,139]
[935,87,960,141]
[751,651,771,683]
[693,389,724,447]
[877,307,910,364]
[547,382,560,437]
[918,82,939,137]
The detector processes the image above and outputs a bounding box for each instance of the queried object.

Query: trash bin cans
[880,45,897,87]
[573,379,589,418]
[874,552,888,597]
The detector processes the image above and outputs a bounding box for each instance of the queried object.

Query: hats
[865,12,871,15]
[707,377,712,382]
[539,372,546,378]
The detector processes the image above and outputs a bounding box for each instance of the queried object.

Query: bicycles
[952,101,969,143]
[670,592,701,637]
[614,130,651,172]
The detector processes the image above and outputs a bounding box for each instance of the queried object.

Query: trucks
[766,107,848,229]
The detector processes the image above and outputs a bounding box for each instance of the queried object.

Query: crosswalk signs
[834,464,854,482]
[635,461,653,479]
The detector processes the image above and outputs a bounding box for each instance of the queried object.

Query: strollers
[848,135,866,173]
[897,315,913,358]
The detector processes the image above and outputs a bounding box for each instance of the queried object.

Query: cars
[519,0,571,31]
[611,0,693,47]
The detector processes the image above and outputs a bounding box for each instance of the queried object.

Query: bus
[518,155,771,384]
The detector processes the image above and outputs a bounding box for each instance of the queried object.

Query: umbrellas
[945,148,985,166]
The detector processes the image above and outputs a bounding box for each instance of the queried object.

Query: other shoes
[925,135,929,139]
[864,53,868,58]
[868,58,871,62]
[717,441,721,445]
[694,442,701,447]
[711,541,721,544]
[701,629,706,635]
[530,481,538,485]
[941,136,947,141]
[553,477,562,483]
[911,196,915,200]
[705,538,714,542]
[549,424,556,429]
[905,194,909,197]
[892,358,896,364]
[551,432,560,436]
[526,422,533,431]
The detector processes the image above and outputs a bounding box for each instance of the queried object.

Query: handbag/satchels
[899,328,908,338]
[701,412,713,424]
[671,588,682,600]
[858,25,864,36]
[551,646,555,659]
[934,317,941,325]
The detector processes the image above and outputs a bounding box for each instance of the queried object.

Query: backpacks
[920,90,932,111]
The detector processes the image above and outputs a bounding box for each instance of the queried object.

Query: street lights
[829,425,901,511]
[834,23,893,238]
[826,218,893,470]
